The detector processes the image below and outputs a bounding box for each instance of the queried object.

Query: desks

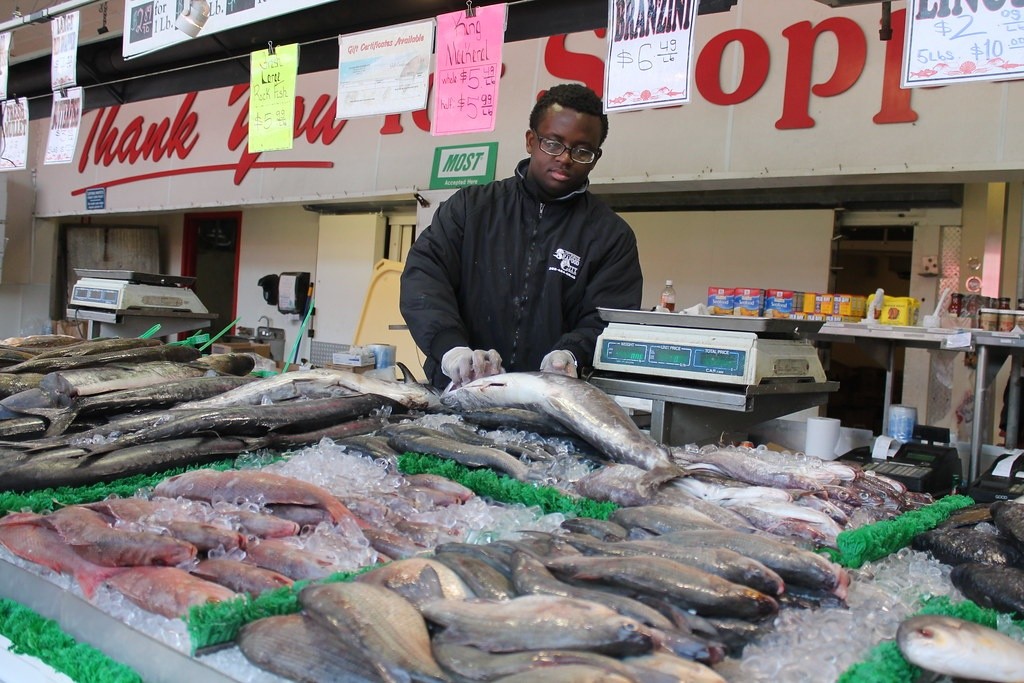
[586,323,1024,495]
[66,305,219,340]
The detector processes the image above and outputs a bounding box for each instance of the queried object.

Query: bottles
[948,293,1024,328]
[660,280,675,312]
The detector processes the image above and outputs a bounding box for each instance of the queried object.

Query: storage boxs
[708,287,919,325]
[212,343,271,359]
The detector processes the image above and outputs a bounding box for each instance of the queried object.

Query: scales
[593,305,829,388]
[69,268,209,314]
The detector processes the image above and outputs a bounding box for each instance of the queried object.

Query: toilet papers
[805,416,852,461]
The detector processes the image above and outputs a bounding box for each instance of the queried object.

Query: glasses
[532,129,598,164]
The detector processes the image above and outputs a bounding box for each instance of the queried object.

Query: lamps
[174,5,209,39]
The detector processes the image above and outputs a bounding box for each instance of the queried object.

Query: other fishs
[0,334,1024,683]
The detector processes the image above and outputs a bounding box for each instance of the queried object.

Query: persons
[397,85,645,391]
